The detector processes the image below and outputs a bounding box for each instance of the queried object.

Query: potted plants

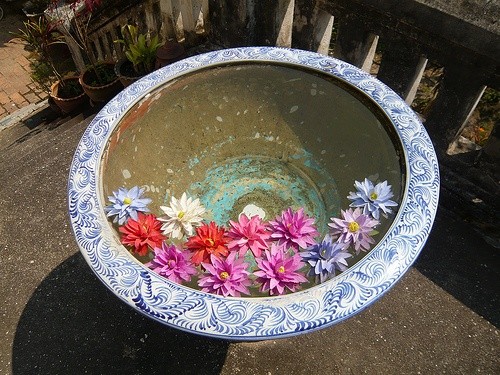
[112,23,164,87]
[11,14,88,114]
[44,1,122,104]
